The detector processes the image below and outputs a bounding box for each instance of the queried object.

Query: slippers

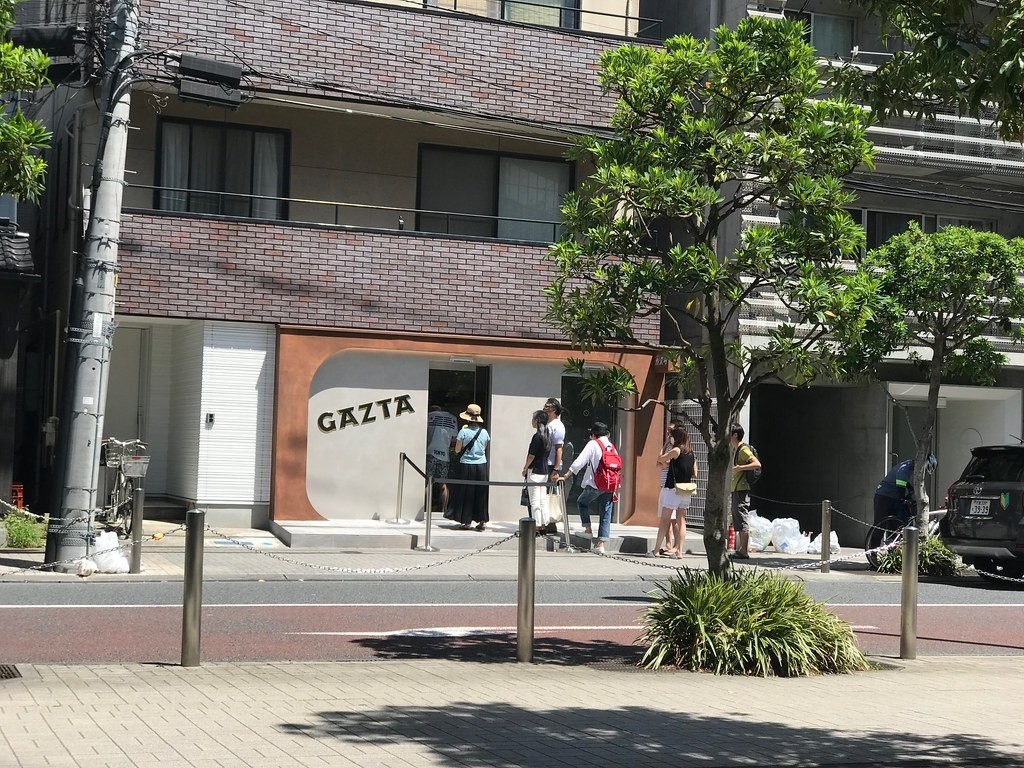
[728,550,749,559]
[669,552,682,558]
[645,551,661,558]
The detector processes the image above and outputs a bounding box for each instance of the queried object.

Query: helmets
[927,452,937,475]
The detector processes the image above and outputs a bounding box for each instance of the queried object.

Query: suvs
[937,432,1024,580]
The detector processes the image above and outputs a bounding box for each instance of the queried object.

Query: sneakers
[591,547,605,554]
[575,531,593,539]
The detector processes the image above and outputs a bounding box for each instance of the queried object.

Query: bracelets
[563,473,569,478]
[553,468,559,471]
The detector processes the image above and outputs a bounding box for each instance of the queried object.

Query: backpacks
[588,438,622,491]
[735,443,761,485]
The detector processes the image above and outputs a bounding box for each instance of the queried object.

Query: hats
[588,422,606,434]
[460,404,484,423]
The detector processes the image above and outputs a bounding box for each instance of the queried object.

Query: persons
[455,402,490,531]
[541,396,566,537]
[555,422,621,555]
[866,452,936,573]
[522,409,552,534]
[727,422,762,561]
[640,419,699,560]
[425,402,456,511]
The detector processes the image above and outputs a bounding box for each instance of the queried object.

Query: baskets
[104,443,151,477]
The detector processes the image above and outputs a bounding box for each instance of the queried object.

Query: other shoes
[459,525,473,530]
[536,528,546,535]
[545,525,557,533]
[476,522,485,530]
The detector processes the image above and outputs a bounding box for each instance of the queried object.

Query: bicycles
[102,437,153,535]
[864,499,972,574]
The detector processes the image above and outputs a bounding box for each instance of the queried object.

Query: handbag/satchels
[520,474,530,506]
[675,483,697,496]
[453,452,460,466]
[547,481,563,523]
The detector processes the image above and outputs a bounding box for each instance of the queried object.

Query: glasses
[544,405,552,409]
